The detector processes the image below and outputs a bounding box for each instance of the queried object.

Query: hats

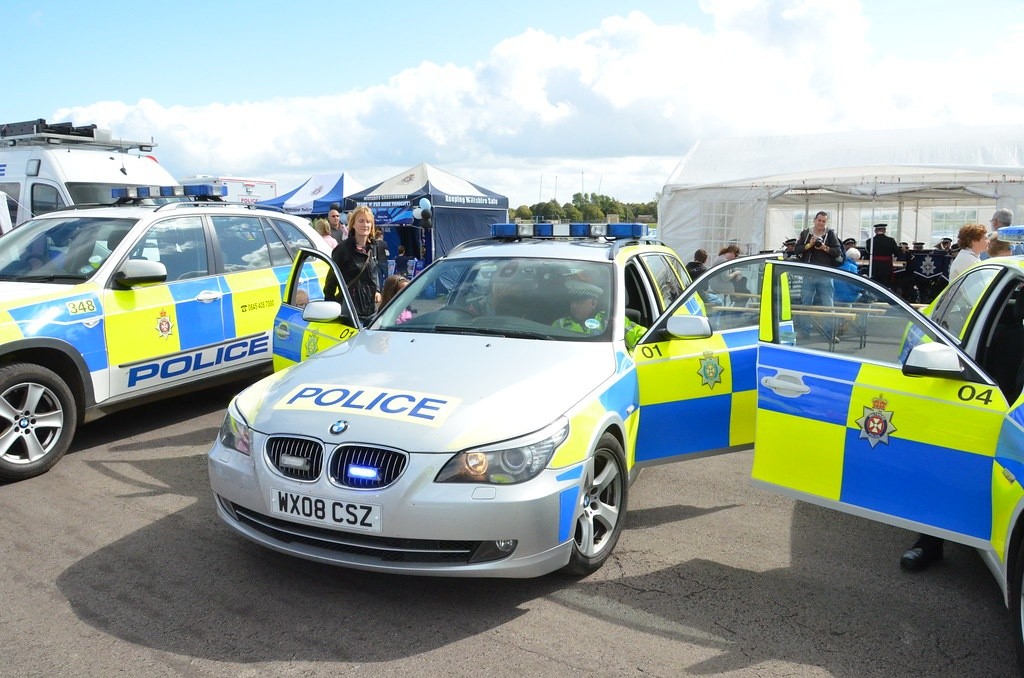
[913,241,925,248]
[564,280,603,301]
[843,237,856,246]
[874,223,888,230]
[785,239,796,246]
[941,237,952,244]
[951,244,961,252]
[900,242,909,248]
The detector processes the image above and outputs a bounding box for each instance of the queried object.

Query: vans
[0,118,197,273]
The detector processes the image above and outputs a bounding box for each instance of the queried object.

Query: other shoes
[827,336,839,343]
[797,331,808,339]
[901,538,944,570]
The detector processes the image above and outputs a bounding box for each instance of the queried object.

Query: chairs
[108,229,129,250]
[464,267,543,318]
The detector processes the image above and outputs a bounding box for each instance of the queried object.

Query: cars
[0,194,336,484]
[752,223,1024,675]
[204,218,784,584]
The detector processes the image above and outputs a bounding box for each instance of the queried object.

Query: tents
[347,162,509,296]
[657,164,1024,294]
[253,172,365,216]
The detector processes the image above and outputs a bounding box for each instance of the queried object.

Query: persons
[554,281,646,354]
[866,224,925,288]
[936,238,959,257]
[949,209,1024,283]
[395,246,409,277]
[323,207,381,327]
[296,289,309,309]
[685,245,751,317]
[314,210,352,248]
[901,535,945,568]
[375,230,390,291]
[784,212,868,313]
[380,274,412,324]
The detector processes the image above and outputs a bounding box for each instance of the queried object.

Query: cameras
[814,237,824,247]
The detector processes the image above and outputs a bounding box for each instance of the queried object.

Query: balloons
[413,198,432,227]
[330,203,347,224]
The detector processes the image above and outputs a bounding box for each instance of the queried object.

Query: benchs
[703,291,930,353]
